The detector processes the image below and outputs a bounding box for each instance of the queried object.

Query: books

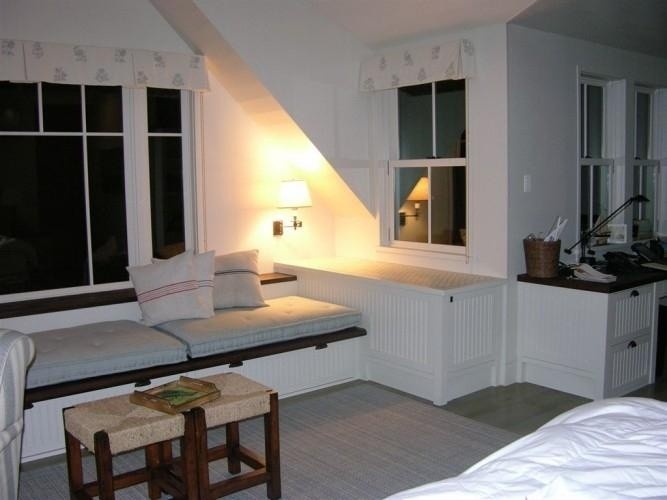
[633,219,652,241]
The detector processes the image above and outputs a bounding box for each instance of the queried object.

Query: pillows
[124,247,270,329]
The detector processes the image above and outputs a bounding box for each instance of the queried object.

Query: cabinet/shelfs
[514,263,666,403]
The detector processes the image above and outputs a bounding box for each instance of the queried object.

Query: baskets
[523,234,561,278]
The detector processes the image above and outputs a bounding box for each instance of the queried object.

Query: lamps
[271,179,314,238]
[398,176,432,226]
[562,193,649,270]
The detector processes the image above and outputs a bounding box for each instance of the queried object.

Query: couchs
[0,325,37,499]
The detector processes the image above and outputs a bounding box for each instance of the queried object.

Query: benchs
[6,295,368,465]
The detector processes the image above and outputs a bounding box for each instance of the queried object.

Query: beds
[381,394,667,500]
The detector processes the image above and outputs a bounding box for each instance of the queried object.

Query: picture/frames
[605,223,627,245]
